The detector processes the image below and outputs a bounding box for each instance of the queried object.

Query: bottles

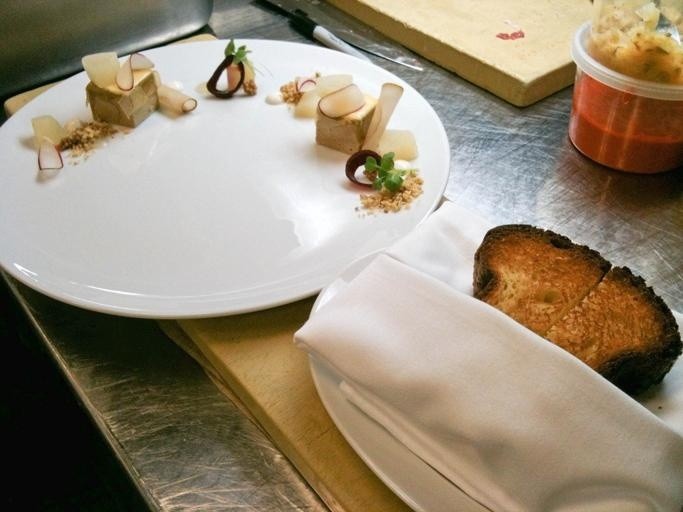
[568,18,683,173]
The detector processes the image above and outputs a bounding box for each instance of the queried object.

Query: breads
[473,224,682,393]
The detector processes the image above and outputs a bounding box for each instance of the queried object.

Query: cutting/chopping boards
[325,0,613,110]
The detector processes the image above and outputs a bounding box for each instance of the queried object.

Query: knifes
[269,1,427,72]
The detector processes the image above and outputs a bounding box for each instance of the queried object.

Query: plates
[0,37,453,325]
